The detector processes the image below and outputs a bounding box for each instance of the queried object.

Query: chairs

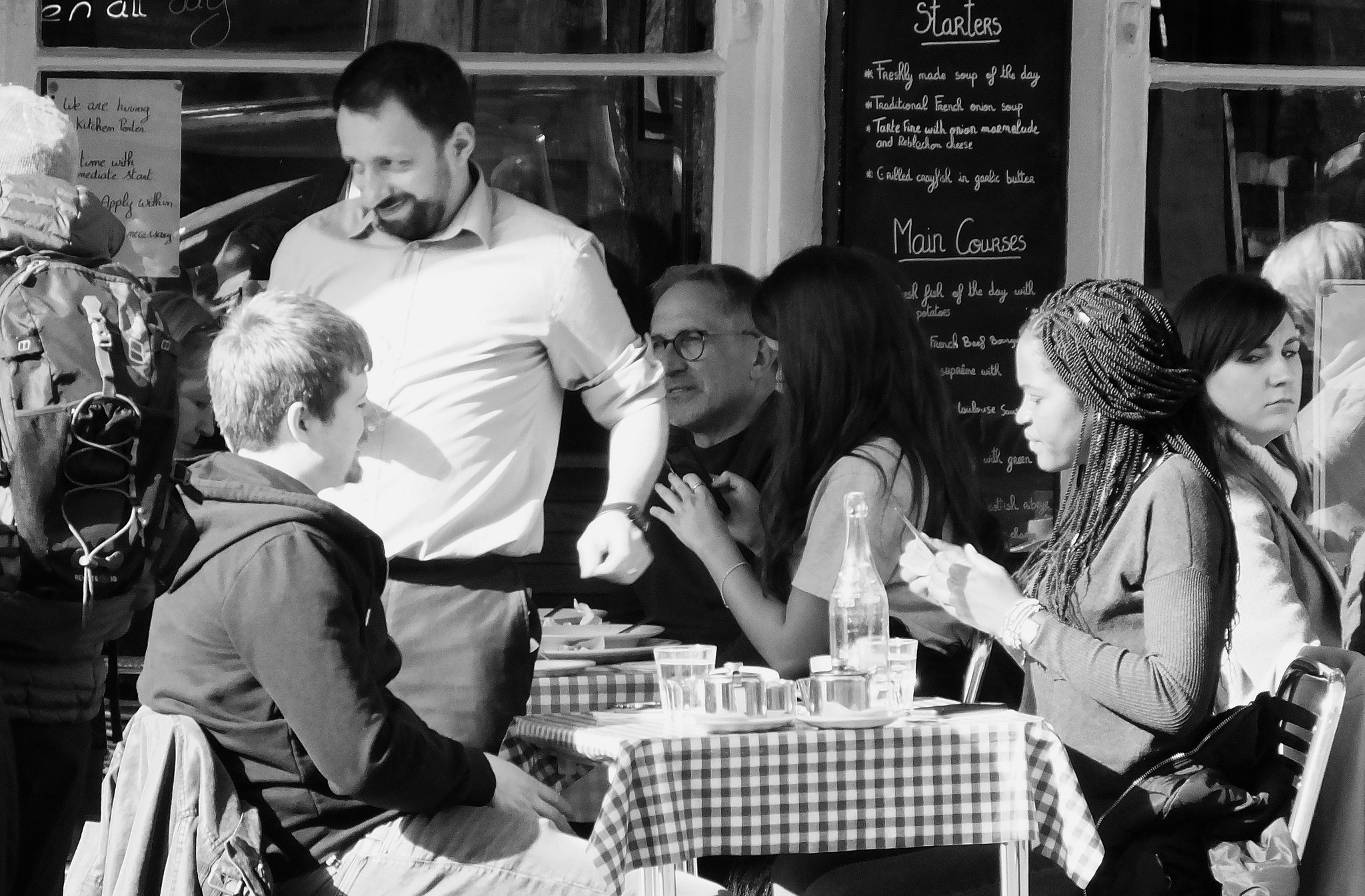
[135,705,215,896]
[1275,645,1365,896]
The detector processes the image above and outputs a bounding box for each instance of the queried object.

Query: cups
[796,675,903,715]
[705,665,797,718]
[890,639,918,710]
[653,644,717,731]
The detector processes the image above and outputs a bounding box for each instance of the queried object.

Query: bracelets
[1000,594,1043,651]
[719,562,754,609]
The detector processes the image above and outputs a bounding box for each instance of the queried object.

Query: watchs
[1018,609,1053,655]
[593,502,653,542]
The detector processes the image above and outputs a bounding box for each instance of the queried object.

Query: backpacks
[0,252,180,601]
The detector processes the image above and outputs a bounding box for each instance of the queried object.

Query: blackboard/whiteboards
[823,0,1072,554]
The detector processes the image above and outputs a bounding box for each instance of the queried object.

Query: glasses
[652,329,762,359]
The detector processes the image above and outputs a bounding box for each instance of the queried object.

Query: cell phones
[896,507,940,555]
[664,440,733,518]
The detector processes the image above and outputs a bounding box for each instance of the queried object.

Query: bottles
[830,491,890,676]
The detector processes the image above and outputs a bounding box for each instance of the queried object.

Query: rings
[691,482,706,493]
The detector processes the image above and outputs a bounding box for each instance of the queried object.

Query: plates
[534,659,596,677]
[698,715,793,734]
[795,714,902,729]
[540,624,665,649]
[537,638,682,666]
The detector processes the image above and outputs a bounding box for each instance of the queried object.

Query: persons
[647,244,1019,896]
[539,264,783,670]
[897,276,1239,896]
[259,41,669,756]
[1166,219,1365,713]
[137,290,625,895]
[0,87,223,896]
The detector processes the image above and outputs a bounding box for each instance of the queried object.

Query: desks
[498,661,1105,896]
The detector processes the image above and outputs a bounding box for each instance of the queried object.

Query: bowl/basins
[537,608,608,625]
